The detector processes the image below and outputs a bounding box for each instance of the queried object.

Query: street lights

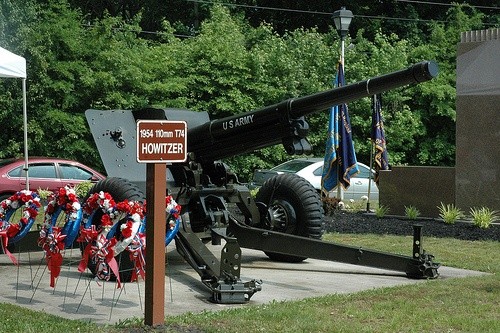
[332,7,354,200]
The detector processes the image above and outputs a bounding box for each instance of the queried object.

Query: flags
[320,61,339,197]
[371,93,391,180]
[337,62,361,191]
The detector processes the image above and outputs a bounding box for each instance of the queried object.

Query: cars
[250,157,379,200]
[0,157,108,203]
